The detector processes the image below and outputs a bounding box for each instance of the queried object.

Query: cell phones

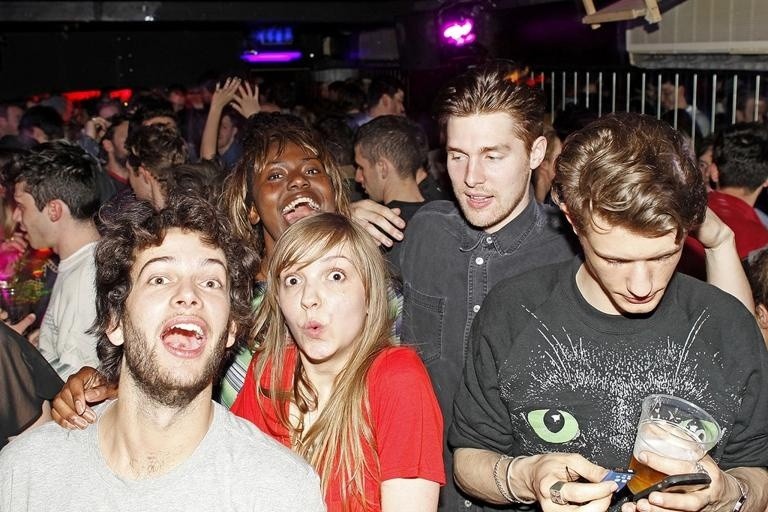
[632,473,712,503]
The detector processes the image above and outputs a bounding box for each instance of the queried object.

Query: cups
[628,392,723,502]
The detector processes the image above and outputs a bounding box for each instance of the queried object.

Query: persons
[0,76,768,512]
